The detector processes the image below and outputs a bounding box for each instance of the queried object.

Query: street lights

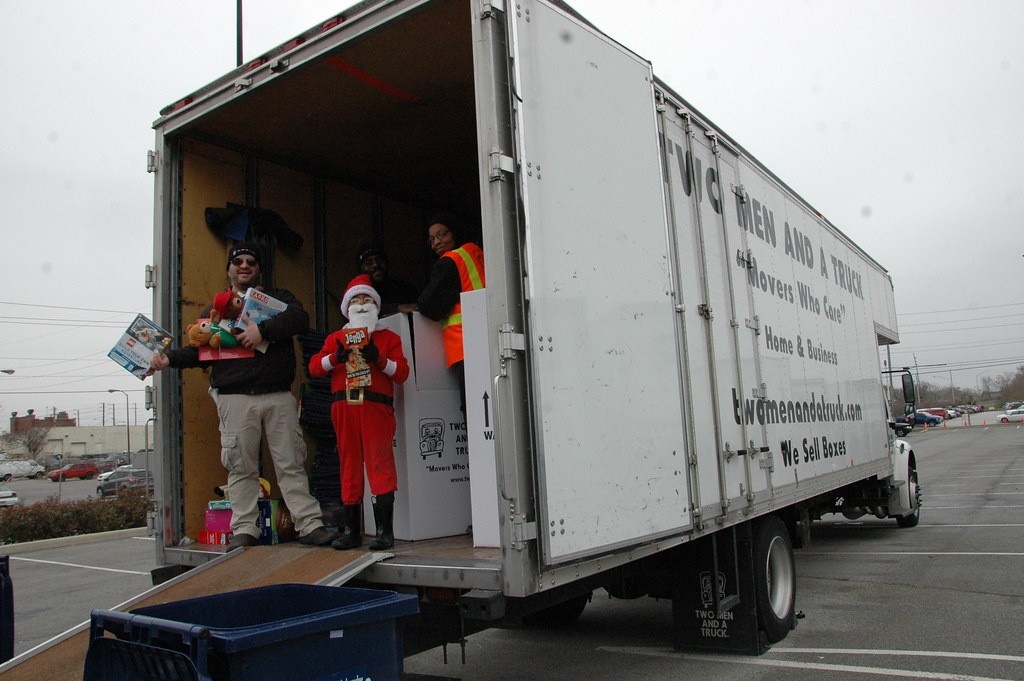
[108,390,130,464]
[976,370,989,405]
[933,376,956,405]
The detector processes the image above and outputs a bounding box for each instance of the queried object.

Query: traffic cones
[925,422,927,429]
[1021,418,1024,424]
[1005,420,1008,424]
[984,420,986,425]
[965,421,967,426]
[944,422,947,428]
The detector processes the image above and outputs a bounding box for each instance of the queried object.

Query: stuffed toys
[186,320,241,348]
[209,289,245,322]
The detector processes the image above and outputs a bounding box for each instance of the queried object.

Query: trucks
[146,1,921,656]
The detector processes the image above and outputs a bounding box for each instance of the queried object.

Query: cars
[917,405,984,420]
[1005,402,1024,414]
[0,485,19,509]
[895,423,912,437]
[996,410,1024,423]
[895,412,943,427]
[96,467,155,498]
[97,464,132,481]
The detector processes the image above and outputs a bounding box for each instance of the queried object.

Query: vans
[0,459,46,482]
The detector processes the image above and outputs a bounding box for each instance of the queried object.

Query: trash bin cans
[83,583,420,681]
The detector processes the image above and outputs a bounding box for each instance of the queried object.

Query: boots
[369,492,394,549]
[331,504,361,548]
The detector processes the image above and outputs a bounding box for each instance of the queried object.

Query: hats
[341,274,382,319]
[227,243,258,271]
[355,243,388,273]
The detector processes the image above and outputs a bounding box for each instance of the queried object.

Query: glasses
[427,229,450,245]
[230,258,258,266]
[351,297,374,304]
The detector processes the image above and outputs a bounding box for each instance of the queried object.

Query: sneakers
[226,533,257,552]
[299,527,337,545]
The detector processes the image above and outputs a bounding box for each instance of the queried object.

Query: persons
[147,242,338,552]
[417,214,487,414]
[356,243,418,317]
[310,274,410,551]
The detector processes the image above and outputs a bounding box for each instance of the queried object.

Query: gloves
[336,339,353,363]
[359,339,378,363]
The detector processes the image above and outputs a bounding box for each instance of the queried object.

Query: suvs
[47,462,99,483]
